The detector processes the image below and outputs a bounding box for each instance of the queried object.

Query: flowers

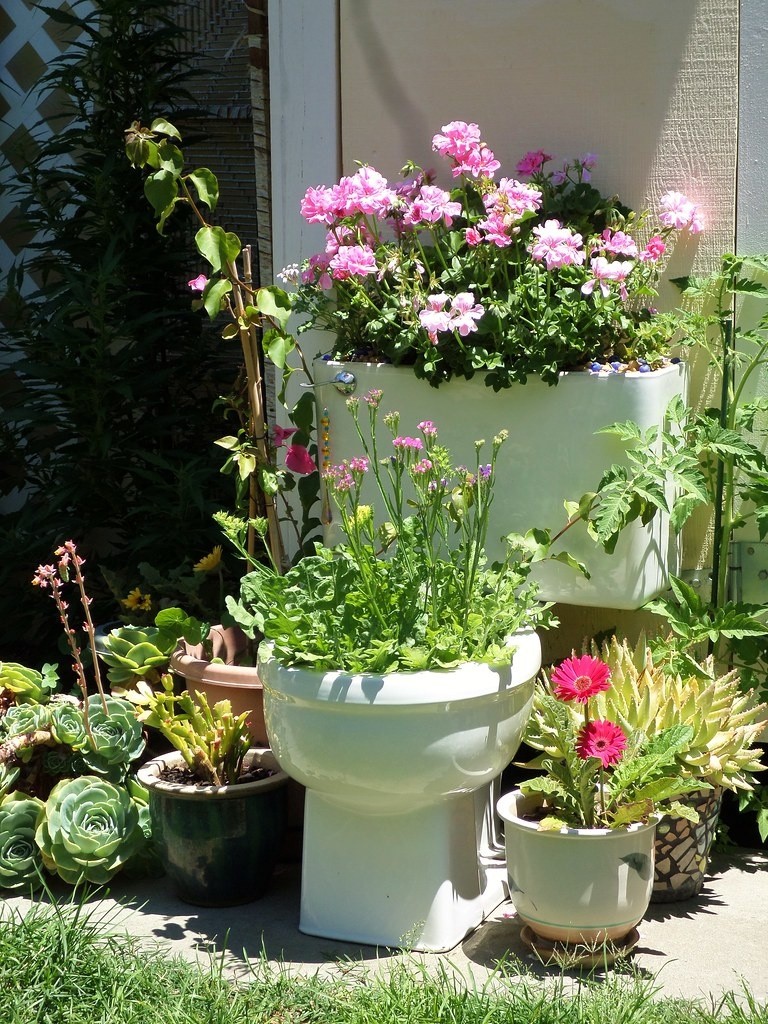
[153,691,258,790]
[523,656,692,831]
[285,122,704,387]
[252,387,548,679]
[527,636,767,817]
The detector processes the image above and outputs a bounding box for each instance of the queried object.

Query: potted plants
[173,613,270,749]
[314,344,689,613]
[497,776,663,972]
[648,773,729,910]
[257,613,542,962]
[137,744,298,917]
